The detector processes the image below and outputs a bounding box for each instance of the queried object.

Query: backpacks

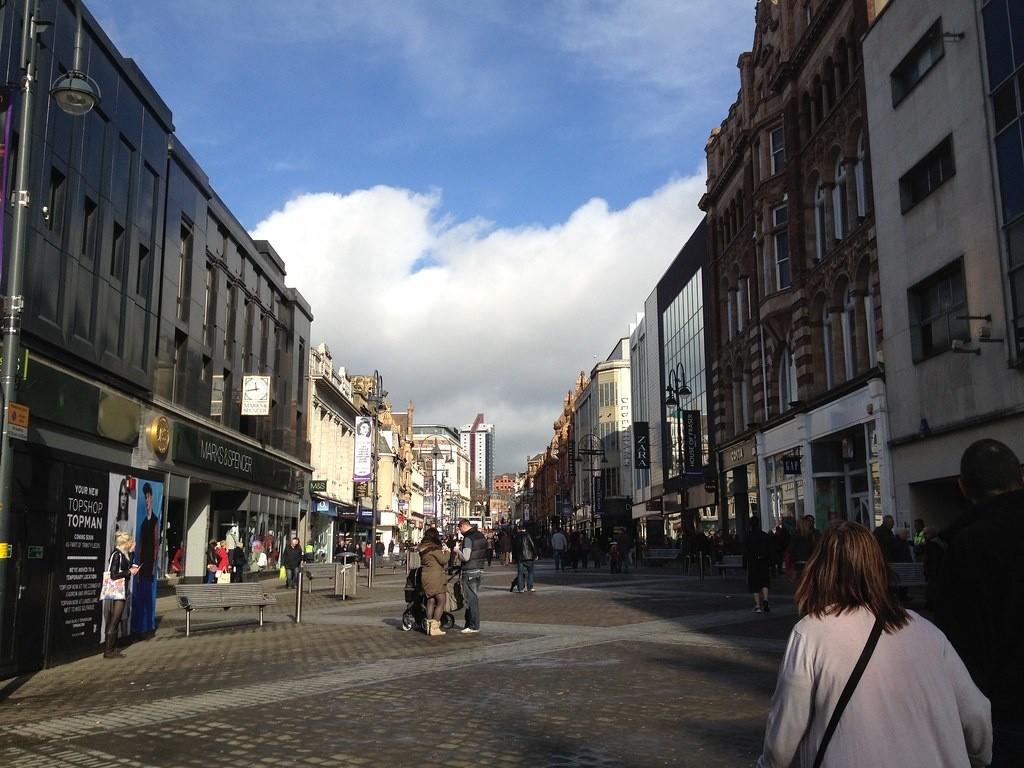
[452,580,464,611]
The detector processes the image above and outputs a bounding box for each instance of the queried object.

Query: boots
[104,624,126,658]
[426,619,447,636]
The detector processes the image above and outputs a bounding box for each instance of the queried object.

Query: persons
[282,538,302,589]
[786,516,822,613]
[111,479,133,637]
[454,519,488,634]
[137,481,158,632]
[443,534,464,576]
[676,514,945,611]
[757,521,994,768]
[930,439,1024,768]
[103,532,139,658]
[740,517,772,615]
[206,539,246,583]
[418,527,452,636]
[333,538,419,569]
[485,523,644,592]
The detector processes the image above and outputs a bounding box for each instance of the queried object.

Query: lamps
[952,327,1003,355]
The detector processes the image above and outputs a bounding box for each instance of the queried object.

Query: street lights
[667,364,691,559]
[417,433,455,529]
[366,370,388,586]
[0,0,102,610]
[574,434,609,537]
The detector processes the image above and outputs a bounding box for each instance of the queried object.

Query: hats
[143,483,152,494]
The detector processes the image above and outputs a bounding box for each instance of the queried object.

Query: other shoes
[519,586,527,592]
[461,627,479,633]
[287,586,291,589]
[531,586,537,592]
[763,599,769,613]
[293,585,297,589]
[752,605,762,613]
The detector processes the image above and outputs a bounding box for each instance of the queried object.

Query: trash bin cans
[409,546,420,569]
[334,552,359,595]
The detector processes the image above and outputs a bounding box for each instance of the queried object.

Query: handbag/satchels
[98,550,126,601]
[214,570,223,578]
[217,566,232,583]
[278,565,286,580]
[207,564,217,572]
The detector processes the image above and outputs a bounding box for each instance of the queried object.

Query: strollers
[401,566,461,632]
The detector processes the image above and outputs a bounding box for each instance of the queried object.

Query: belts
[462,570,483,573]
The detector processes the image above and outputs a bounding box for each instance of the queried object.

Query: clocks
[242,375,270,415]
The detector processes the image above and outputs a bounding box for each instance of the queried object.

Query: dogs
[510,572,525,592]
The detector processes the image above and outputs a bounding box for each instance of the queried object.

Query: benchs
[304,563,339,593]
[890,563,928,586]
[641,548,681,566]
[175,582,278,636]
[713,556,743,580]
